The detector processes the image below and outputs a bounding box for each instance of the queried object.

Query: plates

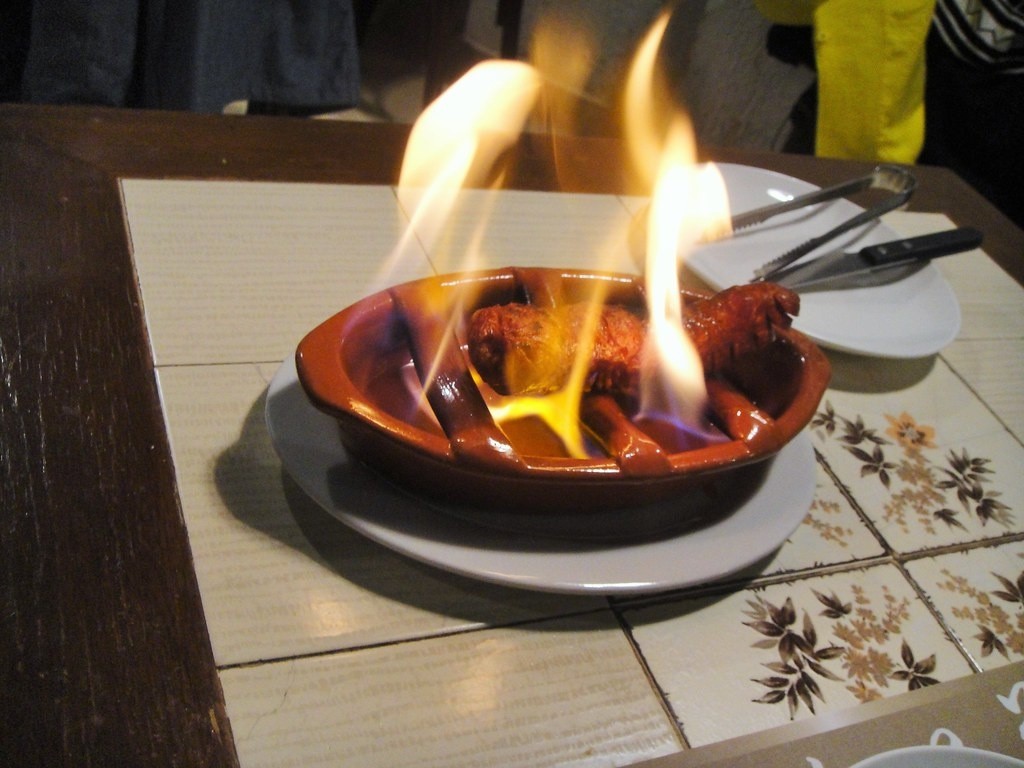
[261,354,818,594]
[685,160,963,361]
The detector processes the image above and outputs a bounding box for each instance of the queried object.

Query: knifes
[765,224,991,290]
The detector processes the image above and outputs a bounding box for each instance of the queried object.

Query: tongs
[668,165,919,285]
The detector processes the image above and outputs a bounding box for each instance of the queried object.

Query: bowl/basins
[296,265,832,509]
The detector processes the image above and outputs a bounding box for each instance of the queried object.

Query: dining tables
[1,106,1024,768]
